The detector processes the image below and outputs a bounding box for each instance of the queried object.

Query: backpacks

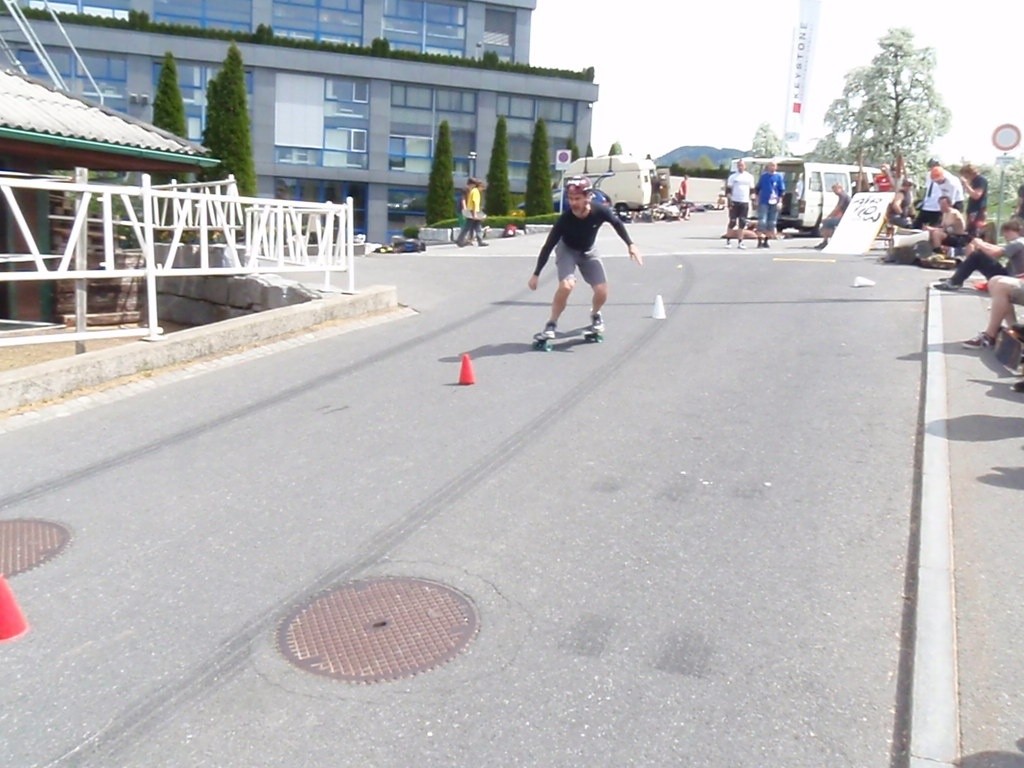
[504,224,515,237]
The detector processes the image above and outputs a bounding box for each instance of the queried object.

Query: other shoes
[455,239,464,247]
[764,243,768,248]
[758,242,762,247]
[465,241,473,246]
[479,242,489,246]
[726,242,731,248]
[815,242,827,249]
[933,277,964,290]
[738,244,745,249]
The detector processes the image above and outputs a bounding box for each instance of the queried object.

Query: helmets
[567,176,592,194]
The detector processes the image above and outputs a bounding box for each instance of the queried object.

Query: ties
[928,182,933,196]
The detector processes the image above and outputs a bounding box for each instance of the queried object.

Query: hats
[931,165,946,182]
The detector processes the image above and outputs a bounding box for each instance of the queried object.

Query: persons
[814,184,851,250]
[725,159,755,250]
[959,273,1024,350]
[672,174,691,220]
[460,177,489,247]
[934,218,1024,292]
[1011,182,1024,217]
[754,160,786,249]
[650,176,663,204]
[886,158,990,254]
[528,176,644,340]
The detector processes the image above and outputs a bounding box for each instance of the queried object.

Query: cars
[516,171,615,212]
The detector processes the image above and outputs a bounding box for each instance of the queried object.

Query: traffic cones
[458,353,476,384]
[650,295,666,319]
[0,574,28,642]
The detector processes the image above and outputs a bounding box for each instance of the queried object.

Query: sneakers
[962,331,995,349]
[543,323,557,338]
[590,309,604,332]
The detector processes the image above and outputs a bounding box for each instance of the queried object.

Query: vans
[561,155,671,213]
[742,156,881,234]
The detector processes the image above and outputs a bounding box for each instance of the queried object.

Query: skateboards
[532,321,607,352]
[993,329,1023,369]
